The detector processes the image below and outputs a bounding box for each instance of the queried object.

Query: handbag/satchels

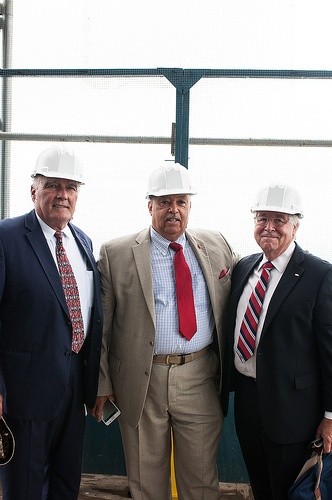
[290,435,332,500]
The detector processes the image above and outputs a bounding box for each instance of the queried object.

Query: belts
[154,348,212,364]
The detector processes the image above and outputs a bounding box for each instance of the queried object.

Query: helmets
[144,162,197,196]
[249,182,305,217]
[29,148,86,185]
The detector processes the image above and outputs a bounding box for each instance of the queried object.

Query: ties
[52,231,86,353]
[236,261,274,363]
[168,242,198,341]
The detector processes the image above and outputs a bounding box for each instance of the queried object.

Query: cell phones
[100,398,121,426]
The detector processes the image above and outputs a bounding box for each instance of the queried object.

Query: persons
[91,162,236,500]
[0,147,102,500]
[222,181,332,500]
